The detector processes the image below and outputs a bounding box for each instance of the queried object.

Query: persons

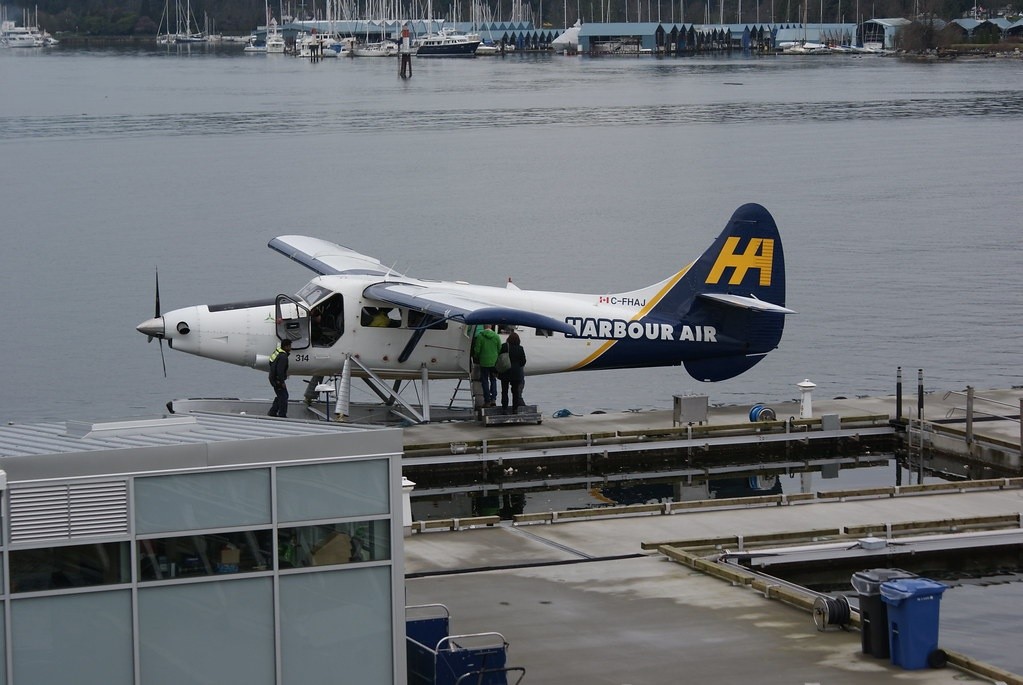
[495,333,526,415]
[362,307,394,327]
[268,339,292,416]
[309,307,321,324]
[467,324,502,407]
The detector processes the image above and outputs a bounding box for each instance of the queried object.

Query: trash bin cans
[849,567,920,660]
[878,577,948,670]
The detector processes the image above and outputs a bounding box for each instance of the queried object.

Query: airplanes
[131,200,801,425]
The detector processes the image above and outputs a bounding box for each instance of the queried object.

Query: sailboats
[0,4,61,50]
[153,0,517,60]
[777,0,908,55]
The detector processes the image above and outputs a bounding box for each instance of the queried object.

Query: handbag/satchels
[495,342,511,373]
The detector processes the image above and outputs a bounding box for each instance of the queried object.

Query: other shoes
[268,411,287,418]
[482,401,490,408]
[512,409,517,414]
[501,409,509,415]
[490,402,496,407]
